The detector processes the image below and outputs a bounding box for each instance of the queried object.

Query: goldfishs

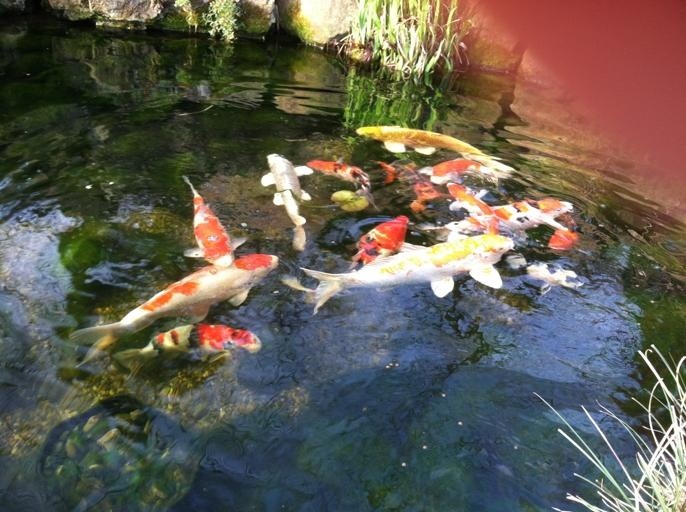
[280,126,583,316]
